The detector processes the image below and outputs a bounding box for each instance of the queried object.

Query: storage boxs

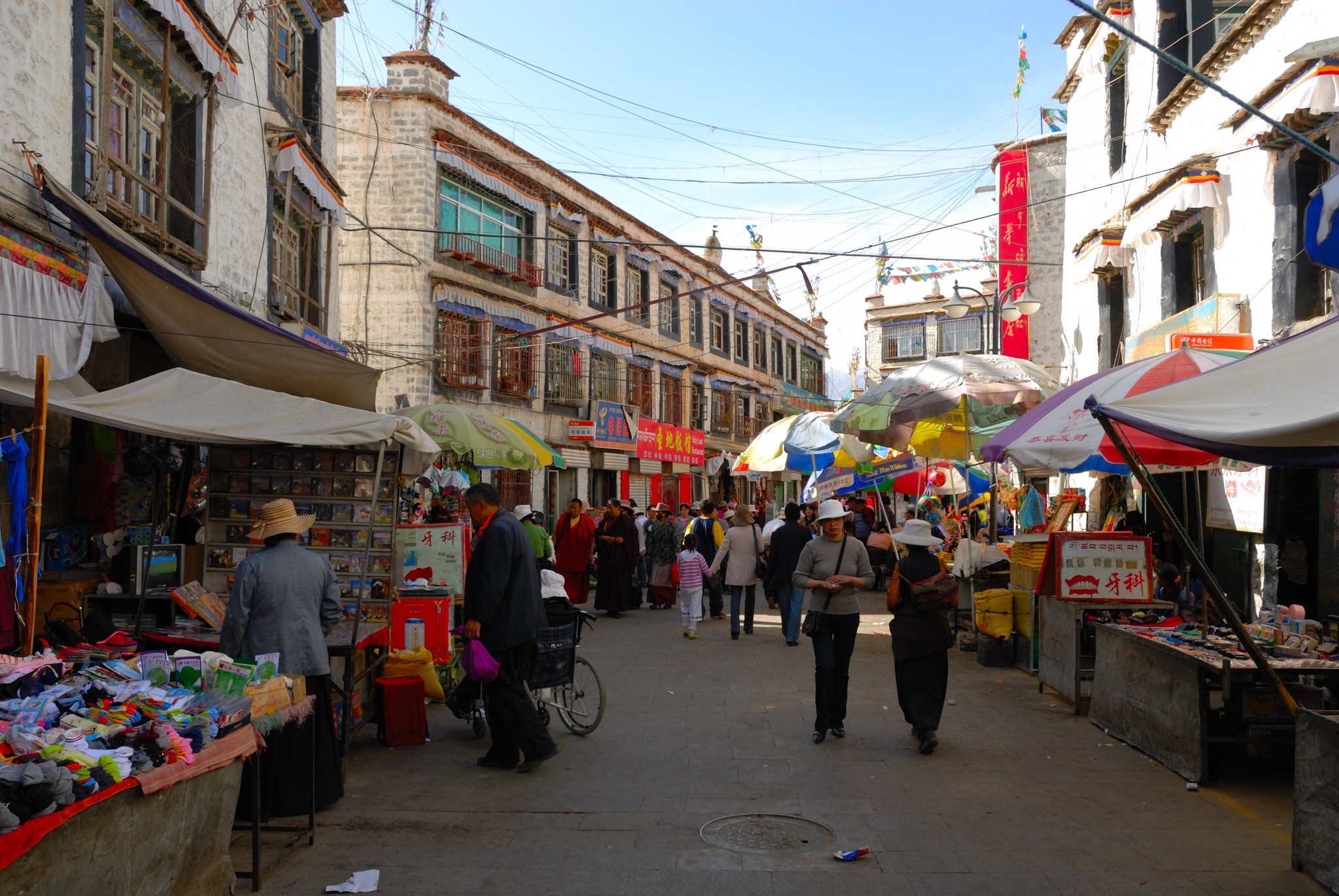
[1274,644,1301,655]
[282,674,307,705]
[329,689,364,738]
[1010,562,1042,590]
[1243,687,1324,755]
[1289,619,1320,634]
[46,524,88,571]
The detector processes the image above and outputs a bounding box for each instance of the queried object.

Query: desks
[80,593,175,643]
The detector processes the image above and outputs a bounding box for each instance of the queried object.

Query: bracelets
[590,551,593,553]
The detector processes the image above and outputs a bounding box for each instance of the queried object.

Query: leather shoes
[831,728,845,737]
[812,730,826,743]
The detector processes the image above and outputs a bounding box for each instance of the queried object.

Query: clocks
[1273,604,1289,625]
[1287,604,1305,624]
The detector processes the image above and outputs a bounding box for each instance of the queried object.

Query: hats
[513,505,534,521]
[246,499,316,539]
[632,507,643,515]
[813,500,854,529]
[540,569,569,601]
[890,519,944,546]
[776,507,786,519]
[651,503,673,516]
[619,499,635,513]
[691,502,700,510]
[848,505,862,512]
[784,498,797,506]
[718,500,737,520]
[730,504,755,526]
[746,504,759,512]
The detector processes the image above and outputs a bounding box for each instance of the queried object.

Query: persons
[218,499,346,821]
[428,483,1194,772]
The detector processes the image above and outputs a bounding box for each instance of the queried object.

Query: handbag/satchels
[448,626,499,681]
[911,573,960,615]
[801,610,822,637]
[671,563,681,586]
[756,555,768,582]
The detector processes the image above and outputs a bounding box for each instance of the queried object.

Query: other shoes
[731,631,739,639]
[911,726,939,755]
[768,597,776,609]
[477,755,518,769]
[518,746,559,773]
[786,640,800,647]
[745,631,752,634]
[710,613,726,619]
[683,628,699,639]
[605,611,622,619]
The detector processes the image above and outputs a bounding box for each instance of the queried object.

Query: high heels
[649,602,673,610]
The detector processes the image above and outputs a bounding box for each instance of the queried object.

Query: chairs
[868,549,890,590]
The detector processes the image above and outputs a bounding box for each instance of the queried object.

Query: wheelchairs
[443,606,607,737]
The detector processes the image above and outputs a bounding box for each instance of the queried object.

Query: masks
[655,512,665,520]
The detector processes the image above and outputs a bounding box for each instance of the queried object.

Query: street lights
[942,272,1043,544]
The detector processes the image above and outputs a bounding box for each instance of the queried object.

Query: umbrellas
[385,403,567,471]
[732,340,1272,544]
